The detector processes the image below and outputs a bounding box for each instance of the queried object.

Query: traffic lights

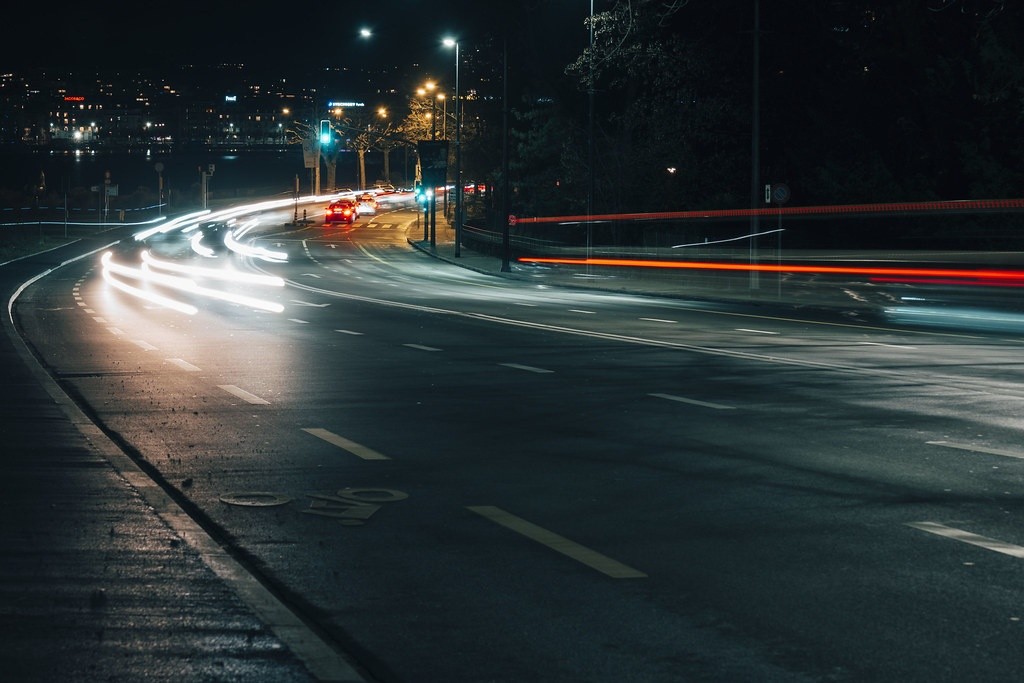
[320,121,330,144]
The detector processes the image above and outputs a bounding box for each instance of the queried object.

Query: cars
[336,199,359,218]
[356,193,377,215]
[324,203,355,224]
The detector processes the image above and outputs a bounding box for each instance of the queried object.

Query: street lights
[418,81,445,245]
[442,38,461,257]
